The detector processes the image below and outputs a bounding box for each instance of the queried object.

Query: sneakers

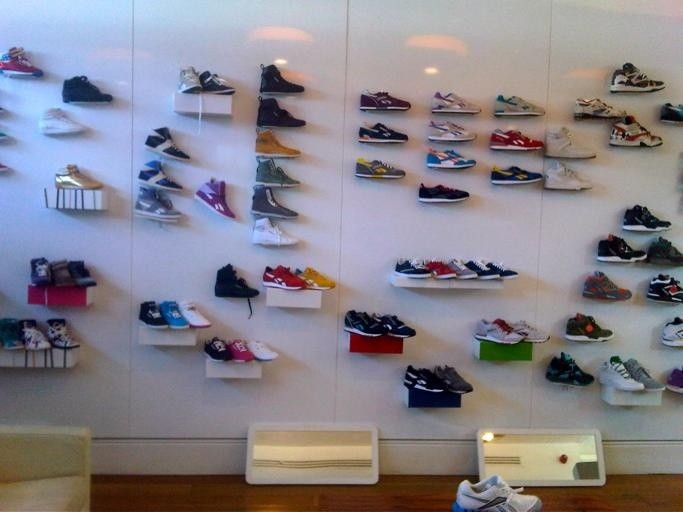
[36,106,87,136]
[472,318,526,345]
[177,66,202,93]
[402,364,449,392]
[486,262,519,278]
[138,301,171,331]
[354,156,406,179]
[358,89,411,111]
[143,126,191,161]
[430,90,481,114]
[563,312,615,344]
[646,236,683,265]
[194,176,237,221]
[608,116,664,148]
[226,338,257,364]
[595,354,645,392]
[425,147,477,170]
[494,93,546,117]
[0,131,7,138]
[427,118,478,141]
[463,258,500,280]
[622,203,673,232]
[449,257,479,280]
[255,159,302,187]
[203,336,233,364]
[29,257,53,287]
[572,96,628,121]
[160,300,192,330]
[69,259,98,288]
[0,45,43,78]
[256,95,307,128]
[488,127,545,151]
[51,259,74,287]
[664,367,683,394]
[544,351,596,388]
[393,257,432,279]
[609,61,665,93]
[660,101,683,124]
[581,271,632,301]
[177,299,212,328]
[261,264,307,291]
[624,358,666,392]
[417,182,470,203]
[596,233,647,262]
[342,309,385,338]
[543,164,593,191]
[46,318,81,349]
[136,159,185,193]
[19,317,52,352]
[0,318,24,350]
[132,185,184,220]
[200,71,237,95]
[249,185,300,217]
[259,63,305,94]
[0,163,9,172]
[660,316,683,349]
[646,274,683,303]
[490,164,544,185]
[452,472,544,512]
[422,257,457,279]
[213,263,260,299]
[370,311,416,339]
[250,216,301,249]
[433,364,474,394]
[246,339,279,361]
[61,75,114,104]
[545,126,598,159]
[295,266,337,291]
[54,163,104,190]
[357,122,409,144]
[254,128,302,158]
[510,319,550,343]
